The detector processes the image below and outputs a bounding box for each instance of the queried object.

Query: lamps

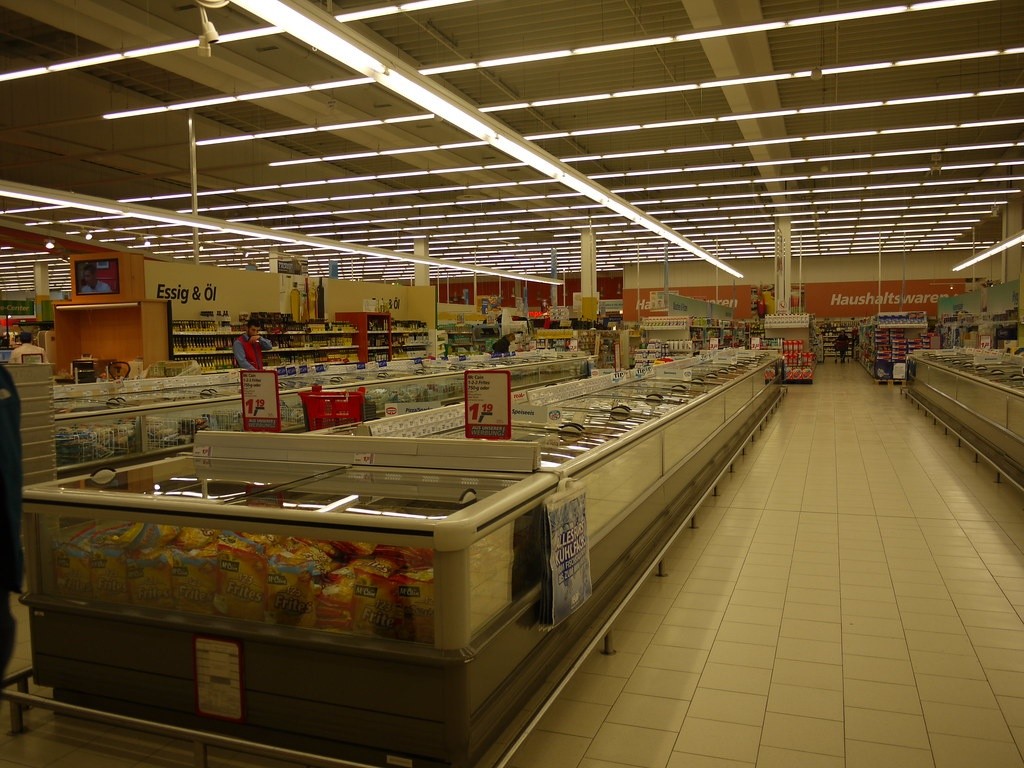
[0,0,745,287]
[951,230,1024,272]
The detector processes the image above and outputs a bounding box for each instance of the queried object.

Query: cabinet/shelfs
[532,329,615,369]
[620,330,643,371]
[438,322,473,355]
[636,316,734,369]
[170,301,429,371]
[823,318,852,356]
[761,314,818,384]
[858,311,928,385]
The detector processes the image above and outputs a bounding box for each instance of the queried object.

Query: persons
[0,364,24,702]
[838,330,850,363]
[492,332,515,357]
[232,318,272,370]
[8,332,48,364]
[82,264,112,292]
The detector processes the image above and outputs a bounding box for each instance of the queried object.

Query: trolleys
[297,383,377,432]
[834,341,849,364]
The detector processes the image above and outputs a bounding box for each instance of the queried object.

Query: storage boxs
[760,338,814,379]
[893,363,905,379]
[978,325,996,336]
[970,331,978,340]
[981,336,993,349]
[997,340,1018,349]
[710,338,718,350]
[751,337,762,348]
[875,361,892,380]
[930,336,942,349]
[964,339,976,348]
